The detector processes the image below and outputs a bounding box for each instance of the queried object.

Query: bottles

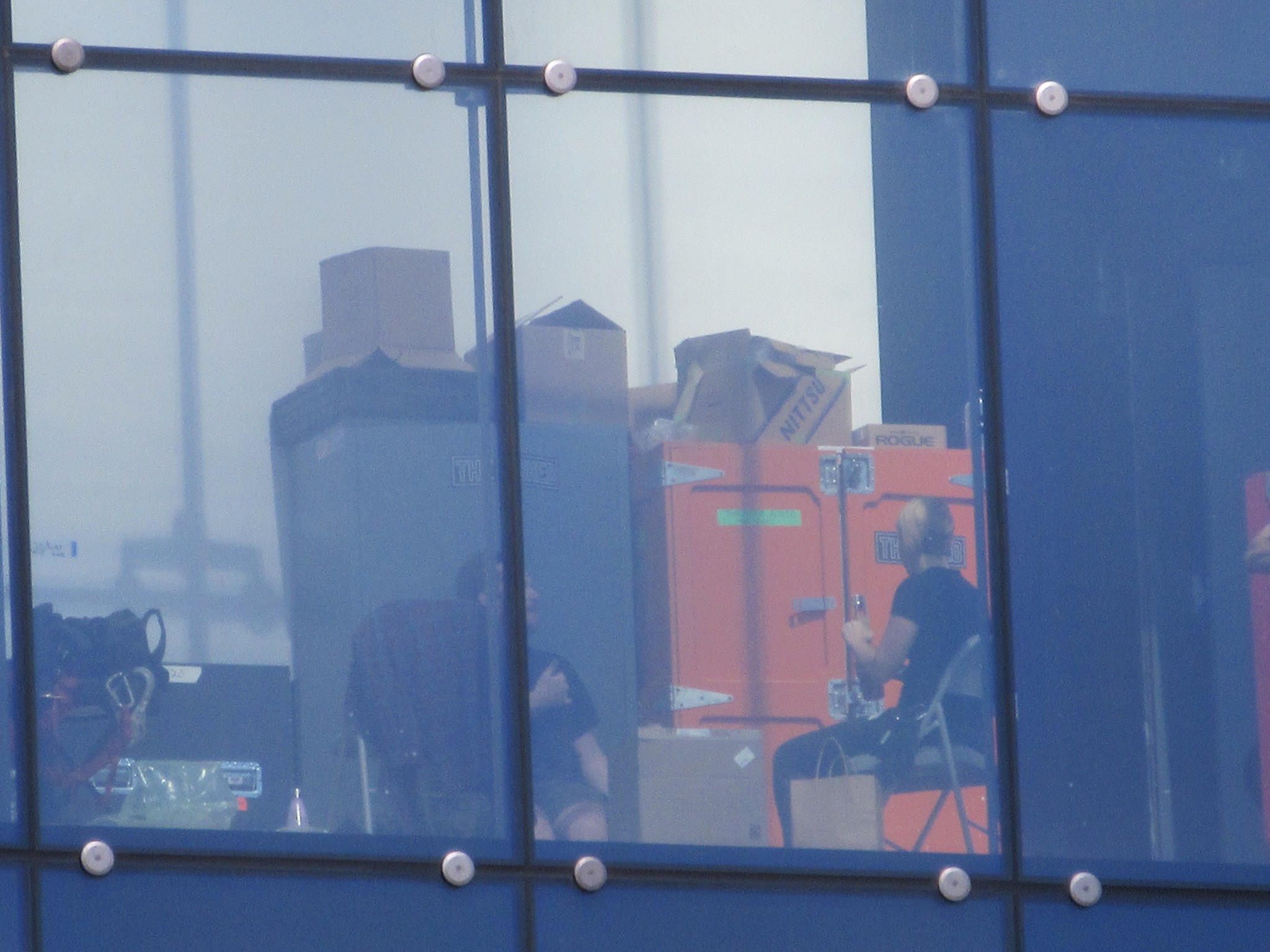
[286,788,309,828]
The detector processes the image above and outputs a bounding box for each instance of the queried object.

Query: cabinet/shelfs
[270,376,640,839]
[631,441,995,857]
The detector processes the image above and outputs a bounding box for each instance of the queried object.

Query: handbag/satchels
[790,734,881,852]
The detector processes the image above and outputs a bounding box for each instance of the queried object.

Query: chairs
[814,632,987,854]
[347,599,493,841]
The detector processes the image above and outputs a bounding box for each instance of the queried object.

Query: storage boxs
[637,725,766,849]
[853,424,947,448]
[303,246,634,427]
[0,665,290,830]
[630,327,853,447]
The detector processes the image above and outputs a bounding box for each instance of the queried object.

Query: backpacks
[28,604,170,827]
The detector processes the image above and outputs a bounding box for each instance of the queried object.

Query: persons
[457,550,610,844]
[772,494,995,848]
